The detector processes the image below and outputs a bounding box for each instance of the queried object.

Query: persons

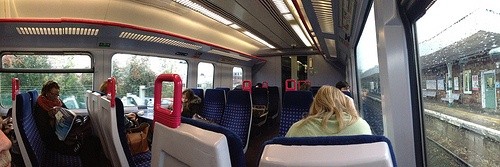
[101,83,150,146]
[285,85,374,137]
[0,130,12,167]
[167,90,202,120]
[336,80,351,91]
[33,81,100,167]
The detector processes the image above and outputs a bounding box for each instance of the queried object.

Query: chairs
[220,90,253,154]
[216,79,280,133]
[11,78,44,167]
[204,89,226,125]
[277,79,314,136]
[151,74,247,167]
[256,135,398,167]
[86,77,152,167]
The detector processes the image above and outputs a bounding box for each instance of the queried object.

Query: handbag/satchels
[124,122,151,155]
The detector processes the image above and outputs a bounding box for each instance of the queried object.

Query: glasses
[50,91,59,96]
